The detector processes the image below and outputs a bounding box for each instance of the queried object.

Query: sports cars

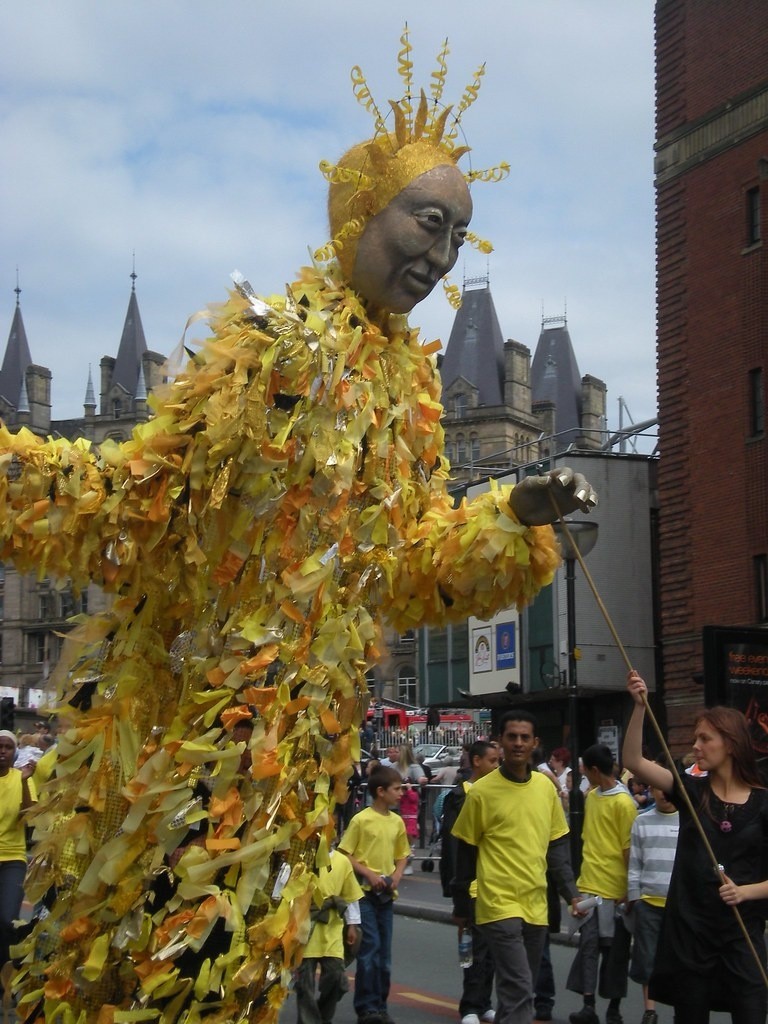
[410,744,459,777]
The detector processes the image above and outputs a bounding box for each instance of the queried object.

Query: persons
[291,807,365,1024]
[342,720,431,856]
[621,671,768,1024]
[0,20,599,1024]
[0,714,70,969]
[430,712,708,1024]
[338,764,411,1024]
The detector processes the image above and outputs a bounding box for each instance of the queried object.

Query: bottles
[457,925,474,968]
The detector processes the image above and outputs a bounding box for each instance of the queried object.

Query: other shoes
[536,1007,552,1021]
[641,1013,657,1024]
[607,1014,624,1024]
[358,1011,394,1024]
[570,1010,600,1024]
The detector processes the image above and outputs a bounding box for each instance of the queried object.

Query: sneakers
[480,1009,496,1022]
[461,1014,480,1024]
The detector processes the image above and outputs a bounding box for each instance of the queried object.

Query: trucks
[365,709,477,737]
